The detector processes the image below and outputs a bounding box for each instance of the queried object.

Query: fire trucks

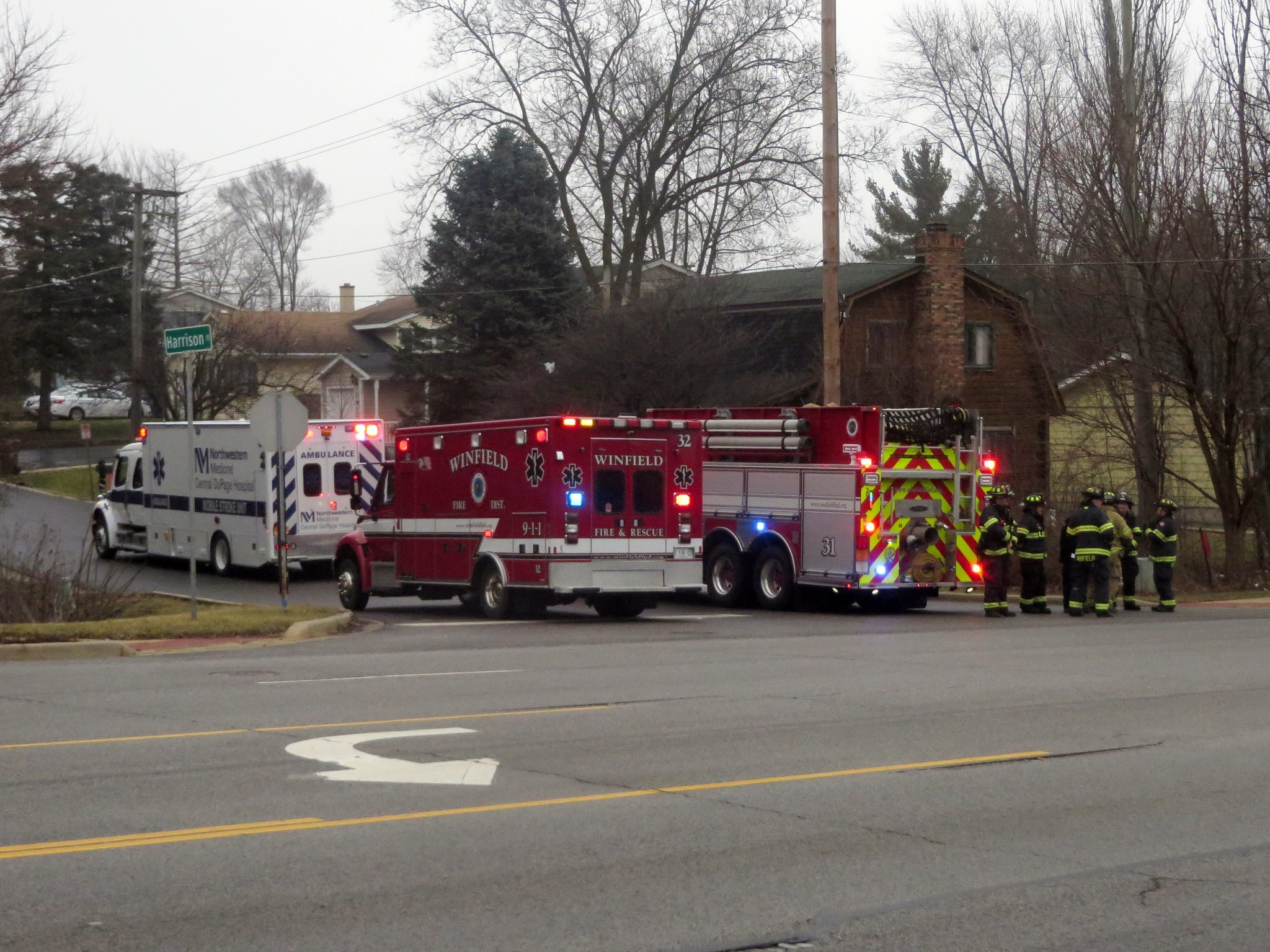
[332,417,705,617]
[643,407,993,611]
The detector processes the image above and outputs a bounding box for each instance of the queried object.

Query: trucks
[92,417,385,580]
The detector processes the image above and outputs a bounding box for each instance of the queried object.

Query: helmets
[1022,494,1045,508]
[1114,492,1135,507]
[1156,497,1177,512]
[986,482,1015,498]
[1081,486,1105,500]
[1101,491,1119,505]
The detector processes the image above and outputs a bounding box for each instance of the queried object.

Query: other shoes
[1124,603,1141,611]
[984,609,1000,617]
[1033,603,1051,614]
[1064,602,1123,617]
[1021,606,1040,614]
[999,608,1016,616]
[1151,604,1175,612]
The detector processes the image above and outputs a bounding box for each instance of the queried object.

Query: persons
[975,482,1019,618]
[1015,493,1054,615]
[1111,491,1144,612]
[1060,500,1091,616]
[1068,486,1114,619]
[1143,498,1179,613]
[1099,491,1135,615]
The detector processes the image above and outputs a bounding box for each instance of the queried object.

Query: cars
[24,383,153,421]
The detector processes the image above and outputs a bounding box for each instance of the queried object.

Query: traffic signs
[164,325,213,355]
